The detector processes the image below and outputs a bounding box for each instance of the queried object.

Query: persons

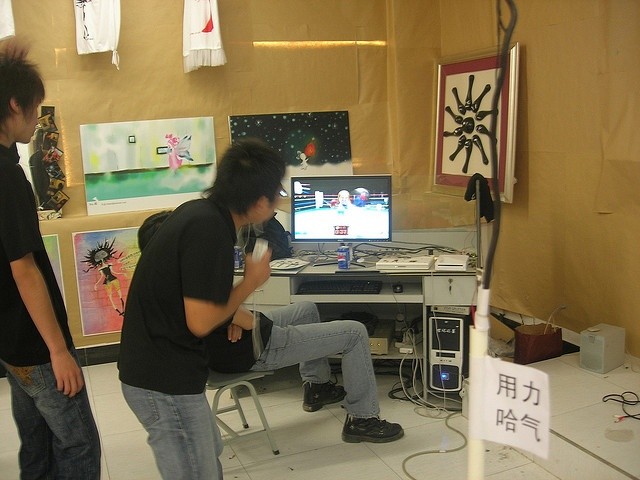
[1,53,101,480]
[117,141,285,480]
[138,210,404,443]
[29,134,54,210]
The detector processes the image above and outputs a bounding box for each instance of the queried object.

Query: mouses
[393,285,403,293]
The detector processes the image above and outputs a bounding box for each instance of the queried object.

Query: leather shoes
[342,413,404,443]
[302,380,347,412]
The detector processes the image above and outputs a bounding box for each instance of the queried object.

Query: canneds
[337,245,350,269]
[233,245,243,270]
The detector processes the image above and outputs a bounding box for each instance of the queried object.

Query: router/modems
[435,255,469,271]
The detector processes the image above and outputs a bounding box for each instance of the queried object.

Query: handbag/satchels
[514,310,563,366]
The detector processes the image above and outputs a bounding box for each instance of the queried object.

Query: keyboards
[295,280,383,294]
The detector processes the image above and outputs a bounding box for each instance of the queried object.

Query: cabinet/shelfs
[232,255,479,407]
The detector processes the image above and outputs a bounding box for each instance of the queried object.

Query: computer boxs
[426,312,474,411]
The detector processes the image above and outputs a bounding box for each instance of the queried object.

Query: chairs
[205,370,280,456]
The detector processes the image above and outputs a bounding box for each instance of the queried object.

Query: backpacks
[243,211,293,262]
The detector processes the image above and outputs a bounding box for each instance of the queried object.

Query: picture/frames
[41,234,65,306]
[73,226,142,337]
[228,110,353,199]
[428,40,521,203]
[80,117,217,216]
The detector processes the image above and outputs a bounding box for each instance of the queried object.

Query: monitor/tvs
[290,174,392,244]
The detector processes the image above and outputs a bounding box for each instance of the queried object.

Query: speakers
[580,323,625,374]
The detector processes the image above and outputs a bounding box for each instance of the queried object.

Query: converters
[400,345,414,354]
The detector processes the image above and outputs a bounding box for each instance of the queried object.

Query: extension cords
[395,342,416,348]
